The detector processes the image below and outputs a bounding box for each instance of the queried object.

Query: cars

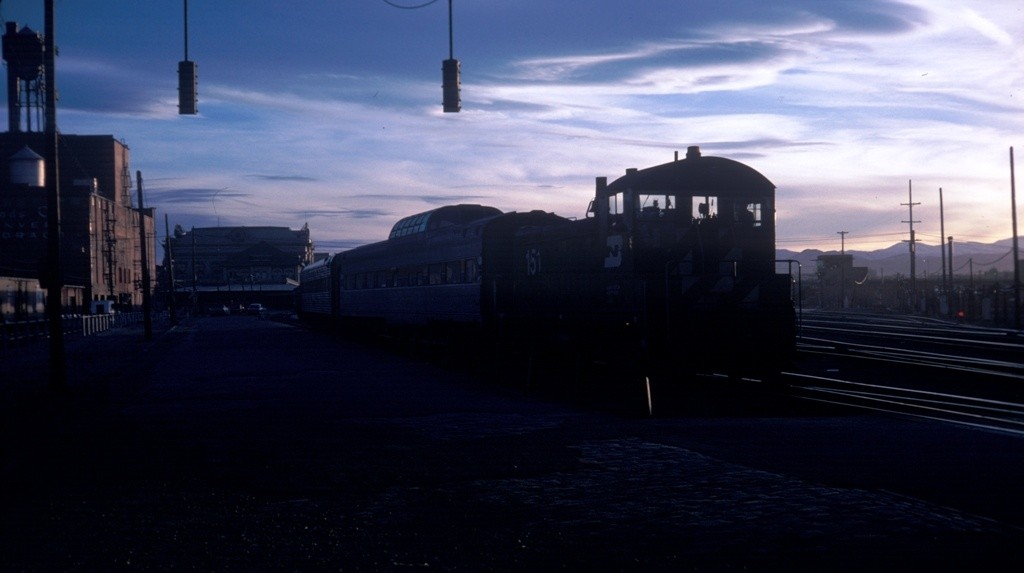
[209,304,233,314]
[247,304,264,314]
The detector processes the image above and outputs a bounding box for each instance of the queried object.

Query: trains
[293,143,803,381]
